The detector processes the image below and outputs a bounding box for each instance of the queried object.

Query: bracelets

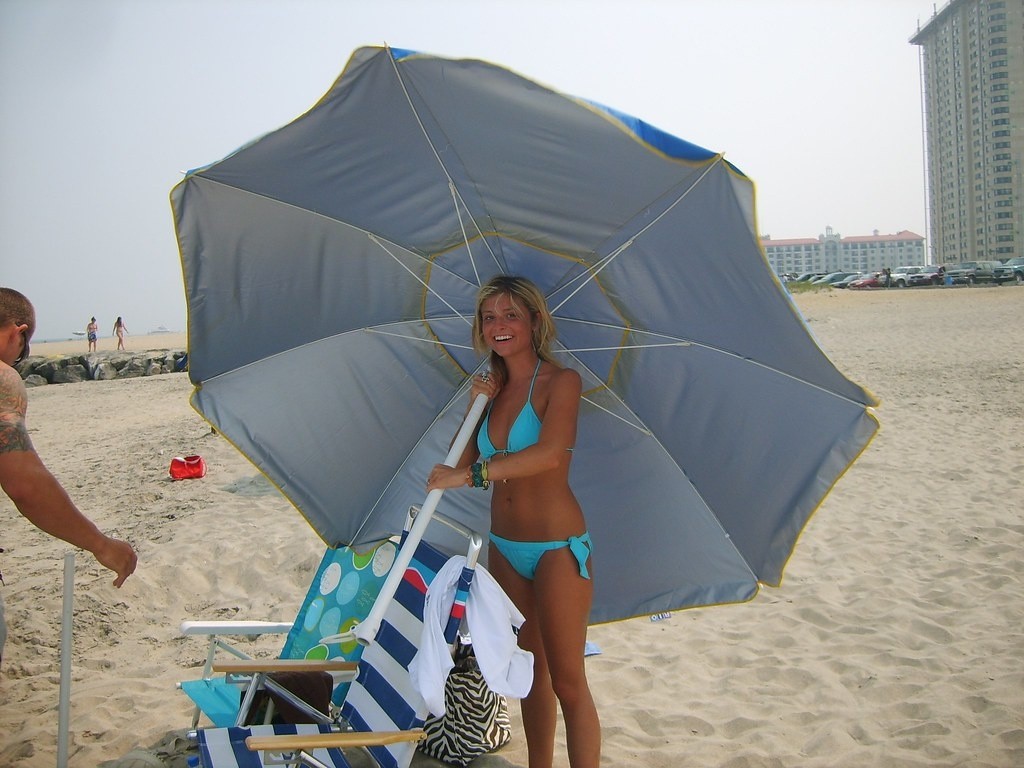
[482,460,490,490]
[465,465,474,488]
[472,463,484,488]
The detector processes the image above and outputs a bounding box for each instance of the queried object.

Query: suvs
[990,256,1024,286]
[938,260,1004,286]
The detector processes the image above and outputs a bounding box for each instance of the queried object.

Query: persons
[0,287,139,664]
[112,316,129,350]
[874,266,892,289]
[425,276,603,768]
[87,316,98,352]
[939,266,946,279]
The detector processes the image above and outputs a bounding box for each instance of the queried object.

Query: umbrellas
[169,42,884,643]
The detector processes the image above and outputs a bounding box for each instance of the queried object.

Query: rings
[482,369,489,377]
[426,479,430,484]
[482,376,489,383]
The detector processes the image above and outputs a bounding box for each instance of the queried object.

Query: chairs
[173,539,401,732]
[185,501,485,768]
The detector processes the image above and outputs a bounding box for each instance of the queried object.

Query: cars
[776,264,959,290]
